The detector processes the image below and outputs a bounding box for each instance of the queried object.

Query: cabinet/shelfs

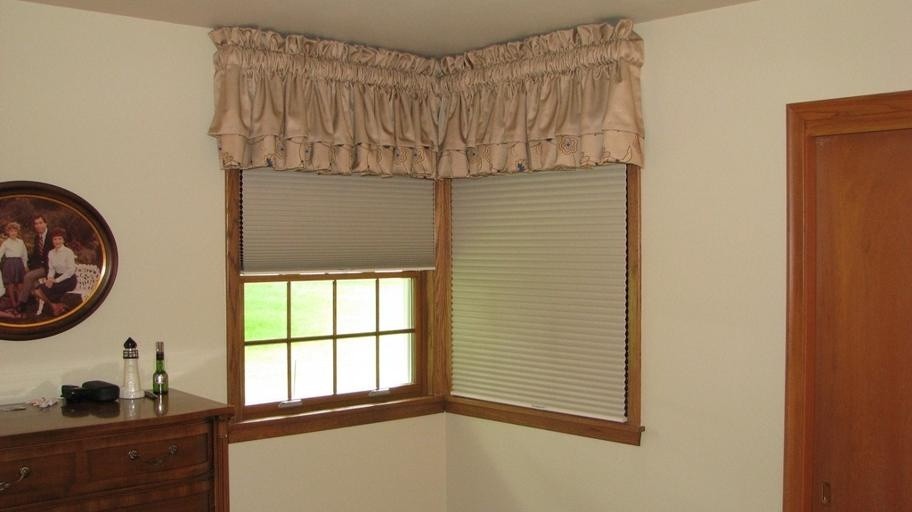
[0,388,235,512]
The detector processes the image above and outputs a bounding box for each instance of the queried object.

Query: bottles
[152,341,169,395]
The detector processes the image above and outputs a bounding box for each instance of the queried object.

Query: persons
[31,226,77,319]
[0,221,29,316]
[10,213,56,314]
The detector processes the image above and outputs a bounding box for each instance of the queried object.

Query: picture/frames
[1,180,118,342]
